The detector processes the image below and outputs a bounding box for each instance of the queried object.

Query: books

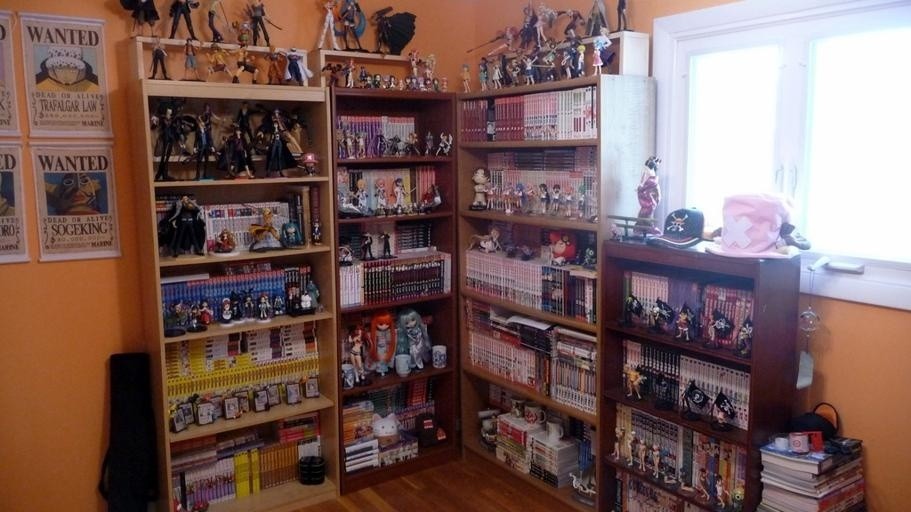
[336,115,416,155]
[755,436,865,512]
[164,320,320,404]
[336,313,375,362]
[339,374,437,474]
[465,221,599,328]
[334,218,453,310]
[618,333,751,433]
[484,145,597,222]
[477,381,597,510]
[456,84,597,139]
[462,294,599,420]
[614,402,746,512]
[604,464,708,512]
[170,408,323,511]
[622,261,755,353]
[158,264,320,327]
[334,163,438,216]
[153,185,323,256]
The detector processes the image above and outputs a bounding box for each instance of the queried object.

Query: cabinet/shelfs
[456,31,657,512]
[597,238,802,512]
[129,37,340,511]
[308,49,461,495]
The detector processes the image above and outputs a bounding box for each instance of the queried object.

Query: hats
[646,208,704,248]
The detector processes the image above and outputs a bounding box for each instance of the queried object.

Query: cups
[395,354,411,377]
[341,363,354,390]
[545,417,563,442]
[789,432,809,454]
[510,398,525,421]
[524,402,546,427]
[431,345,446,369]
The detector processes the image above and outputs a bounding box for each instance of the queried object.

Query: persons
[305,279,320,311]
[481,182,497,210]
[624,430,636,466]
[338,241,354,266]
[471,166,489,208]
[593,41,604,76]
[164,195,206,258]
[739,327,749,357]
[279,223,305,250]
[388,135,401,158]
[435,130,451,156]
[501,180,514,213]
[403,132,421,158]
[587,0,610,36]
[524,184,537,213]
[465,25,521,55]
[541,36,563,80]
[423,130,435,156]
[408,48,420,78]
[147,36,172,80]
[168,0,199,40]
[404,75,413,90]
[576,184,585,218]
[675,310,690,340]
[342,58,356,88]
[339,0,366,52]
[371,73,381,88]
[121,0,159,38]
[205,43,235,82]
[521,55,539,85]
[381,74,391,89]
[417,77,427,93]
[242,288,254,321]
[431,78,442,92]
[310,218,322,247]
[263,44,286,85]
[715,409,727,427]
[707,314,717,348]
[373,178,390,216]
[594,26,613,74]
[397,308,430,374]
[365,74,373,88]
[419,183,442,212]
[611,426,624,461]
[652,443,662,480]
[534,4,558,48]
[336,130,347,160]
[255,295,270,320]
[358,230,377,259]
[166,300,183,330]
[546,227,574,266]
[182,37,203,80]
[229,127,256,180]
[576,46,587,77]
[264,120,297,178]
[391,179,410,211]
[617,0,628,32]
[563,8,585,40]
[150,106,179,182]
[474,224,504,253]
[289,106,311,158]
[228,291,241,320]
[699,468,710,502]
[292,290,312,314]
[425,54,436,81]
[198,103,224,154]
[220,297,233,323]
[503,240,517,260]
[229,41,260,84]
[637,439,647,474]
[625,363,644,401]
[239,19,248,47]
[562,37,581,77]
[582,241,600,270]
[424,78,433,92]
[713,472,727,509]
[247,207,288,251]
[193,123,209,180]
[214,229,237,254]
[359,65,368,87]
[410,76,419,90]
[286,46,305,86]
[246,0,283,46]
[396,76,405,90]
[460,64,472,92]
[344,130,355,159]
[634,157,664,237]
[317,0,341,50]
[322,62,342,87]
[208,0,225,43]
[507,58,520,88]
[624,296,632,326]
[513,183,526,213]
[389,75,396,90]
[535,181,550,215]
[662,463,677,484]
[367,6,416,54]
[173,112,199,157]
[375,128,386,158]
[272,294,285,318]
[186,302,198,327]
[490,59,504,89]
[233,100,263,155]
[477,64,489,91]
[549,183,561,215]
[379,230,393,257]
[214,136,234,180]
[520,5,545,49]
[355,179,372,215]
[481,56,489,65]
[200,300,214,326]
[368,310,396,378]
[346,323,370,383]
[563,185,573,217]
[264,103,299,156]
[296,152,318,177]
[678,468,694,492]
[355,132,368,159]
[651,301,661,331]
[522,243,534,260]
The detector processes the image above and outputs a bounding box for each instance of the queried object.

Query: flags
[687,379,710,411]
[653,296,674,325]
[711,308,732,340]
[714,389,736,420]
[742,313,753,337]
[680,301,698,330]
[655,371,669,388]
[628,293,643,318]
